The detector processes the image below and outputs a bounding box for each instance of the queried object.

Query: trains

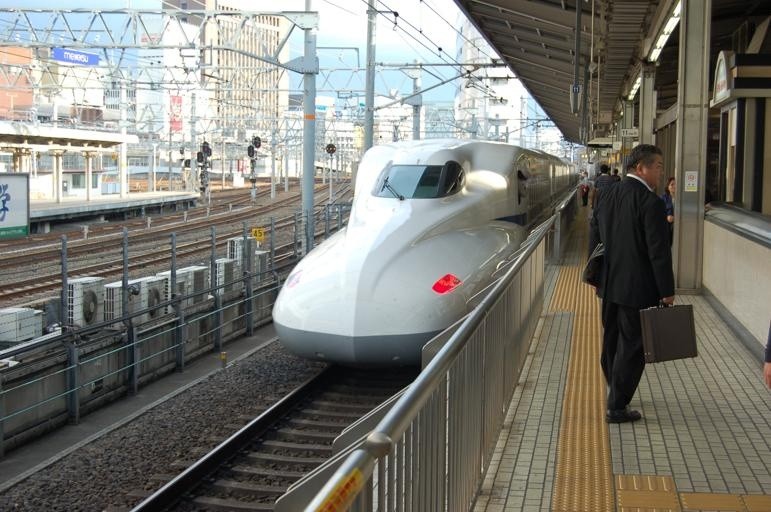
[266,137,582,372]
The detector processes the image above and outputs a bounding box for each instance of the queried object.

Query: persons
[587,144,675,423]
[659,177,676,246]
[580,164,621,211]
[763,320,771,389]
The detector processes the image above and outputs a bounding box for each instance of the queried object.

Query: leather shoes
[606,407,641,423]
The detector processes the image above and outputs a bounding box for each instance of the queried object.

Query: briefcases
[640,300,698,363]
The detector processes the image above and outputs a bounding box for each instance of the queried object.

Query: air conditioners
[60,234,270,346]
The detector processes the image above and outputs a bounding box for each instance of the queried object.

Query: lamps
[624,0,680,104]
